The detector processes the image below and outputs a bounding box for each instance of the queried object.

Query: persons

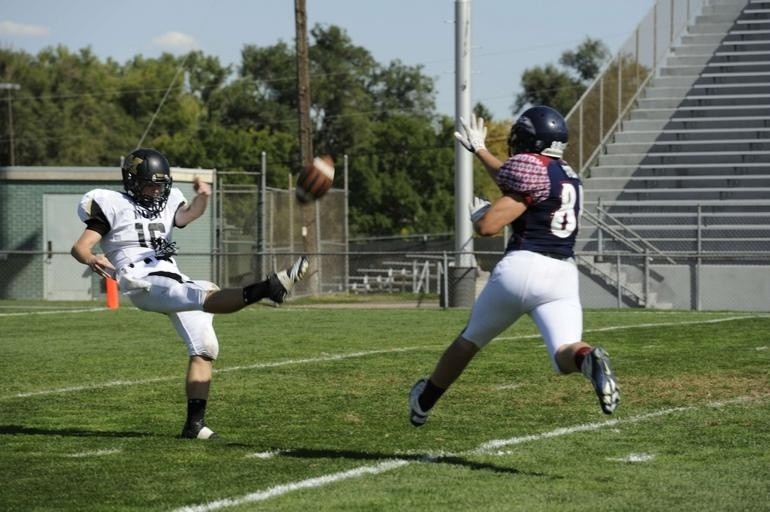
[71,147,310,437]
[407,105,620,427]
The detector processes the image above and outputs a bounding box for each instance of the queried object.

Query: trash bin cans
[441,267,479,308]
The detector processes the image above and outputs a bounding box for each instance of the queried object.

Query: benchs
[572,0,770,264]
[321,261,442,295]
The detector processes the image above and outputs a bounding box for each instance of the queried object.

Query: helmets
[121,148,173,193]
[516,105,569,159]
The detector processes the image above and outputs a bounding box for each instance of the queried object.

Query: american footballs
[295,154,335,203]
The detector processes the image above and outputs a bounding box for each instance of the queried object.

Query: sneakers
[182,419,218,440]
[581,346,621,415]
[409,378,429,427]
[267,255,309,304]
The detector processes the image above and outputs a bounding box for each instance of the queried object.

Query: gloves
[453,113,488,154]
[469,196,493,223]
